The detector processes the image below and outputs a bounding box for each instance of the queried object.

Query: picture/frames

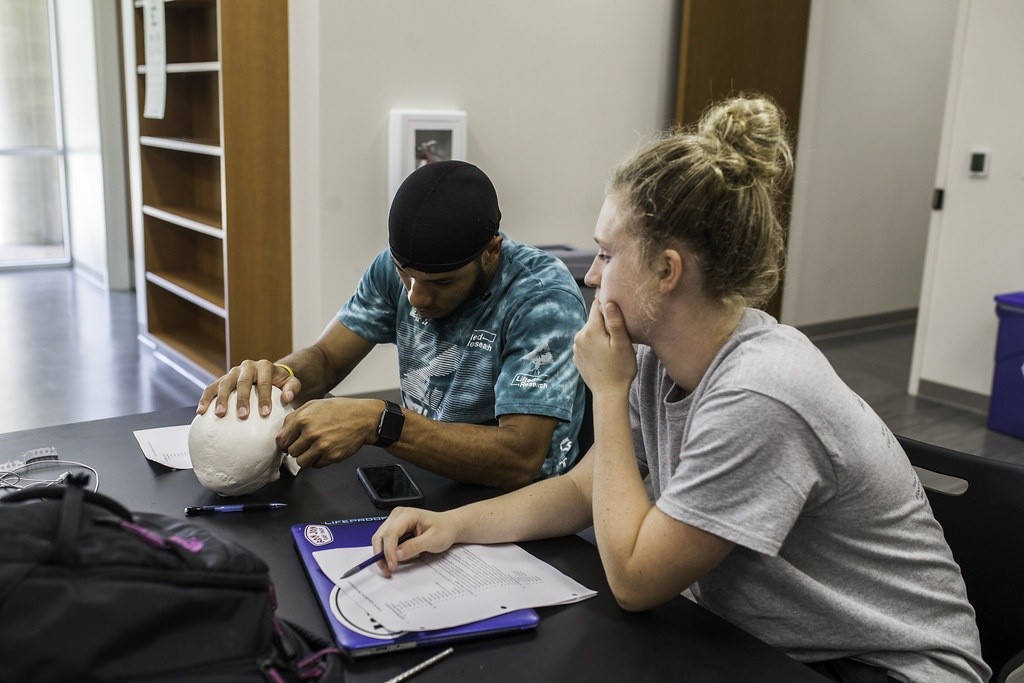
[388,108,468,215]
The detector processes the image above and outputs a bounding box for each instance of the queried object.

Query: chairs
[892,431,1024,683]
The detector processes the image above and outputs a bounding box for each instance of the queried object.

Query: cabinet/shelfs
[132,0,292,382]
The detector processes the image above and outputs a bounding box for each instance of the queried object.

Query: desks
[0,392,834,683]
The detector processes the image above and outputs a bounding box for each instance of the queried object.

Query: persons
[196,161,588,492]
[372,99,994,683]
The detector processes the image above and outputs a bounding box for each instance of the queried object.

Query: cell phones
[357,464,425,508]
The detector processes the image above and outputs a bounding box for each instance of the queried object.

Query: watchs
[372,399,406,449]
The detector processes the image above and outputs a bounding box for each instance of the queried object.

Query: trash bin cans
[985,290,1024,440]
[529,243,600,320]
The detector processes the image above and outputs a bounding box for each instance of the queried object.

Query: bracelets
[274,364,294,377]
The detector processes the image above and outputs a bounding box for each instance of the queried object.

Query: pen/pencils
[373,647,454,683]
[184,501,289,518]
[338,532,413,579]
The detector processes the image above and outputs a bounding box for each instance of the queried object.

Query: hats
[388,159,501,274]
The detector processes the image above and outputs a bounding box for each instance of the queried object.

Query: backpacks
[0,477,342,683]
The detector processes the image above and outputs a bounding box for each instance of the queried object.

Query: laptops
[291,514,540,656]
